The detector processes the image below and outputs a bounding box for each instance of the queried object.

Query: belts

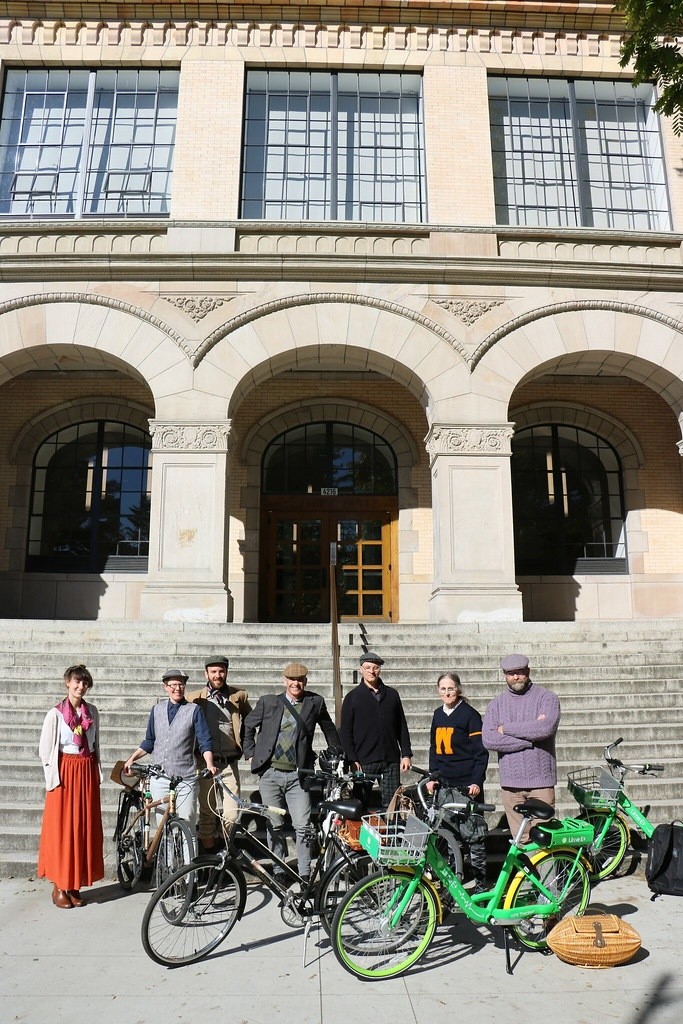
[212,756,239,763]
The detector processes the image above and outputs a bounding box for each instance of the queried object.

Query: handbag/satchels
[385,785,417,822]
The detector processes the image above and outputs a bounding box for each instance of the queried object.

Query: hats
[284,664,308,679]
[500,654,528,672]
[162,670,189,683]
[360,653,385,666]
[205,655,228,669]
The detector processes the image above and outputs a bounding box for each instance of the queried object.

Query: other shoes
[187,883,198,901]
[274,873,287,888]
[162,884,174,898]
[298,876,315,892]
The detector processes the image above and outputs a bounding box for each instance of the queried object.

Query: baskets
[359,810,433,866]
[567,766,623,808]
[399,783,431,822]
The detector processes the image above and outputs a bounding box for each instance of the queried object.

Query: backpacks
[645,820,683,896]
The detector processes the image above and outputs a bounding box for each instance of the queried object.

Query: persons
[244,663,339,886]
[37,664,105,908]
[185,655,253,853]
[341,653,414,808]
[426,671,489,894]
[481,654,561,905]
[124,670,222,903]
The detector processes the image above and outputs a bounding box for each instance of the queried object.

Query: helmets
[319,745,348,774]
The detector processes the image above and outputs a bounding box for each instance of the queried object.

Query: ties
[216,691,224,708]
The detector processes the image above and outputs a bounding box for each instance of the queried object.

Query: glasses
[439,686,457,696]
[507,670,523,677]
[166,684,185,688]
[361,665,379,671]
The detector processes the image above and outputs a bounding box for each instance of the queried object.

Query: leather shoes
[52,883,72,908]
[67,890,86,906]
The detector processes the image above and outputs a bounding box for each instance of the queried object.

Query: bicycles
[330,769,597,983]
[113,762,217,925]
[310,751,472,935]
[557,736,657,885]
[139,772,428,968]
